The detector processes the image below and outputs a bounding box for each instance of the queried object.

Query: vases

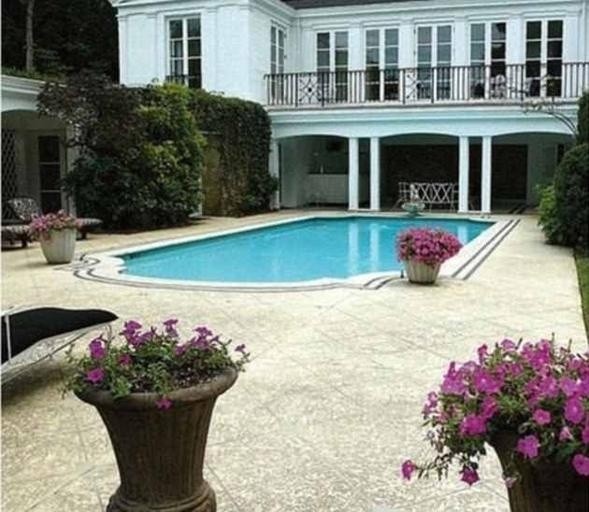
[404,260,440,283]
[487,431,589,512]
[39,228,78,263]
[71,363,237,512]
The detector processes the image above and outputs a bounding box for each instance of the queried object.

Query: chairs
[1,196,104,247]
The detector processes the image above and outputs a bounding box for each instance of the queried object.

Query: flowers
[56,317,255,411]
[395,225,463,269]
[402,332,589,489]
